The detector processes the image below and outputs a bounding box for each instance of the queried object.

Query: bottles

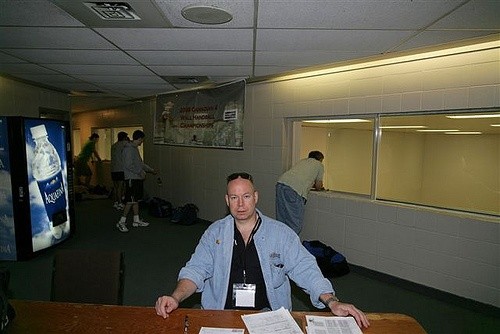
[30,124,70,239]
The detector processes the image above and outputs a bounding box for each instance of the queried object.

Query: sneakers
[113,202,119,208]
[116,221,129,232]
[117,203,126,210]
[132,218,150,227]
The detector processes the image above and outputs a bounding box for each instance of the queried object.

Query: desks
[0,299,428,334]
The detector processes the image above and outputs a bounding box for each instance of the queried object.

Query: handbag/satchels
[147,197,174,217]
[169,202,199,225]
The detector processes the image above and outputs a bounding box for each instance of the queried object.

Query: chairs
[50,248,125,305]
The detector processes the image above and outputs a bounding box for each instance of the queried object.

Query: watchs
[154,173,370,329]
[326,297,339,308]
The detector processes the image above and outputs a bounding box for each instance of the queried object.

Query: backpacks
[303,240,350,278]
[92,183,109,195]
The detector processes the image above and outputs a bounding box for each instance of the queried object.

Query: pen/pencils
[185,315,187,331]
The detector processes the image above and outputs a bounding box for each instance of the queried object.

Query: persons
[275,151,324,236]
[111,132,130,211]
[75,133,101,186]
[116,130,153,232]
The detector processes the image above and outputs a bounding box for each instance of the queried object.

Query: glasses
[227,173,253,183]
